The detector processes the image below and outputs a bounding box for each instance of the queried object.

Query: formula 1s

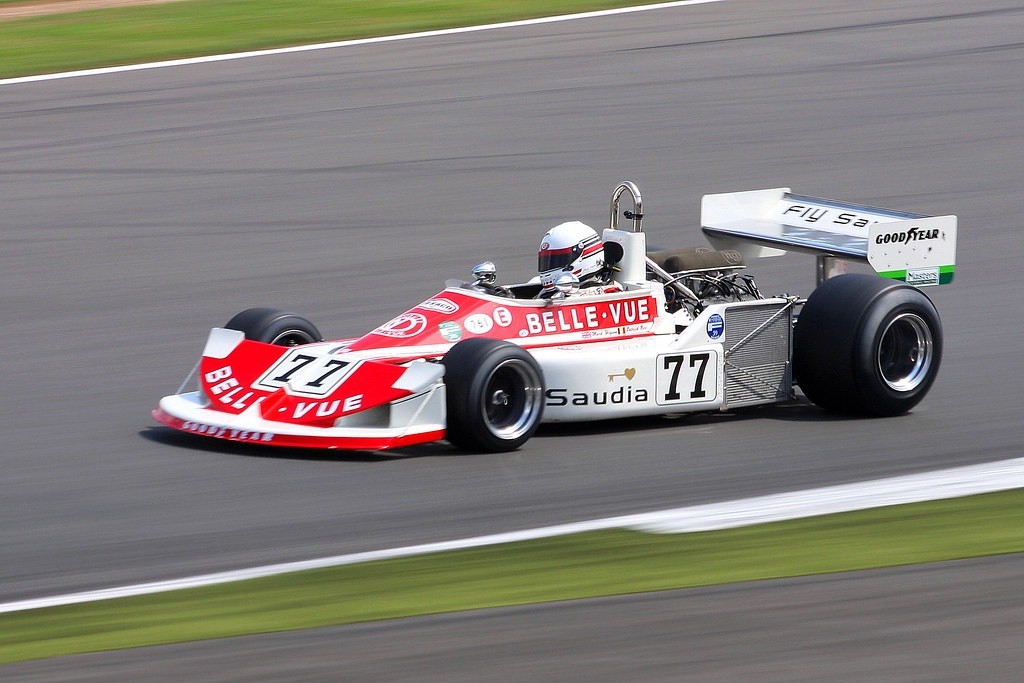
[147,181,960,458]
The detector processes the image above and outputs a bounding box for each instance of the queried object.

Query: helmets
[539,220,604,293]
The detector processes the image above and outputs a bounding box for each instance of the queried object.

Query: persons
[532,221,624,299]
